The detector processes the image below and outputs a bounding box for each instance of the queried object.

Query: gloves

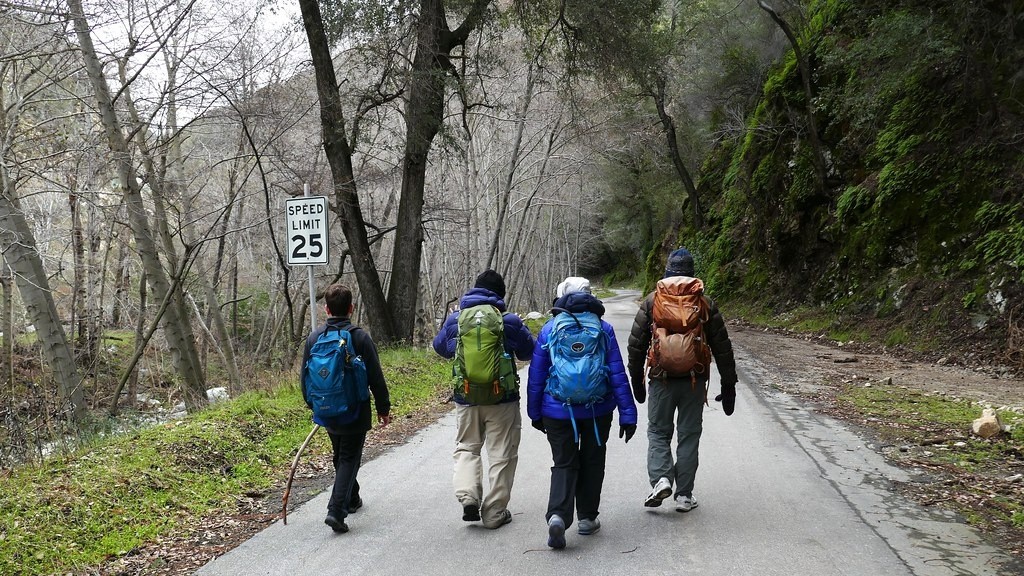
[630,377,646,405]
[532,419,545,433]
[619,423,637,443]
[715,382,735,415]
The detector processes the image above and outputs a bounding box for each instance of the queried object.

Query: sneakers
[325,510,349,535]
[462,502,481,521]
[577,517,601,535]
[644,477,672,508]
[674,494,698,511]
[548,514,566,550]
[501,509,512,526]
[347,498,363,513]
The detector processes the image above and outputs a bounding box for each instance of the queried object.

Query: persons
[300,284,391,534]
[526,277,638,549]
[433,270,538,530]
[627,249,739,513]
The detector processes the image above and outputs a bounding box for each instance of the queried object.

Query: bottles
[504,354,510,358]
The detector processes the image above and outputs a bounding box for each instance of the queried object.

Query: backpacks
[544,310,610,411]
[642,275,711,406]
[308,323,370,418]
[449,303,517,406]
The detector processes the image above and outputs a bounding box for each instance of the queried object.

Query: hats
[557,276,593,298]
[474,269,507,298]
[665,248,696,279]
